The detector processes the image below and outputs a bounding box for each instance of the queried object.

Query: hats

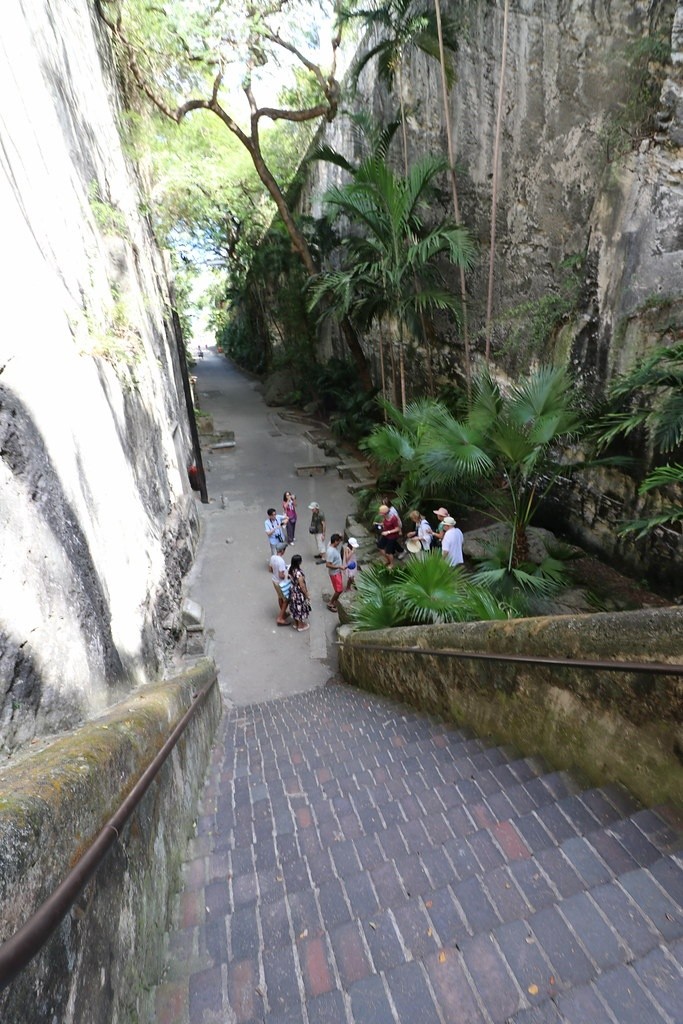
[378,505,389,515]
[407,539,422,553]
[433,508,450,517]
[441,517,456,525]
[346,562,356,570]
[276,543,288,551]
[348,538,359,547]
[308,502,319,509]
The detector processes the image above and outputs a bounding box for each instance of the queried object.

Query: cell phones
[281,523,284,526]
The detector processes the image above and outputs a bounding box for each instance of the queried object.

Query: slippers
[277,622,291,626]
[284,612,290,620]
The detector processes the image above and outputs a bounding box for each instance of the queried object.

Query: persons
[263,490,467,633]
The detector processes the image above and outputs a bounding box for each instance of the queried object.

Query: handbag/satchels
[279,572,292,599]
[289,514,297,523]
[309,527,318,534]
[430,539,442,550]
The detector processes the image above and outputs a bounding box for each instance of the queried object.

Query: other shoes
[316,560,326,565]
[298,624,309,631]
[290,543,294,545]
[293,538,296,541]
[327,603,338,612]
[398,549,406,559]
[293,625,297,629]
[314,554,322,558]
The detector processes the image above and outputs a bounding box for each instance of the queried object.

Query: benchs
[209,441,236,455]
[294,461,328,476]
[337,462,370,479]
[348,479,377,495]
